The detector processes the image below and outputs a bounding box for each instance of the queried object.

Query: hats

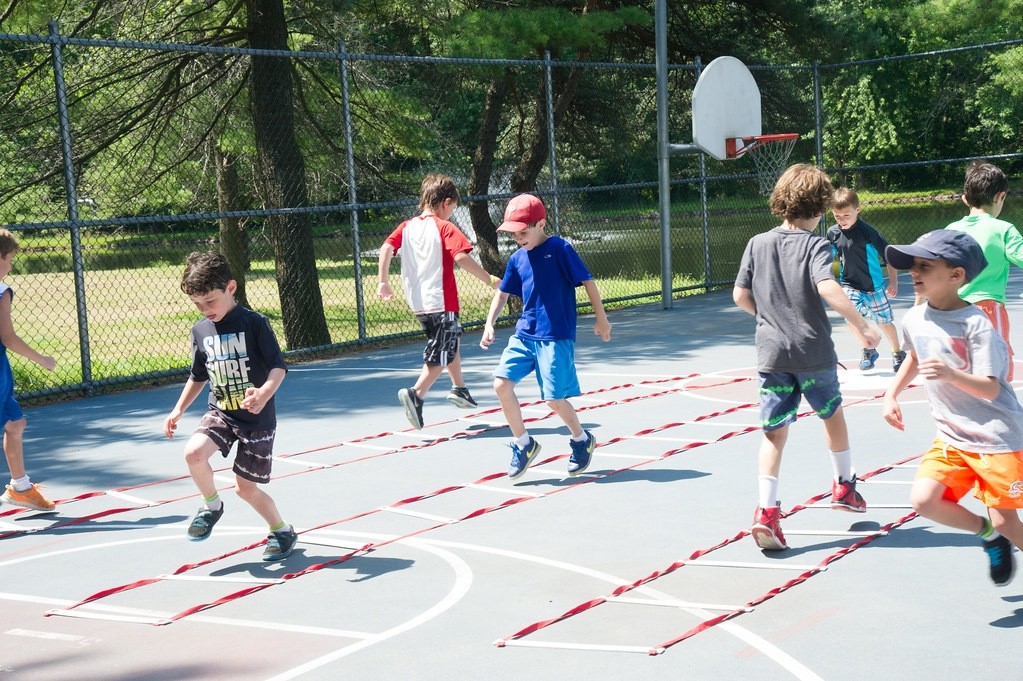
[496,193,546,232]
[885,229,989,283]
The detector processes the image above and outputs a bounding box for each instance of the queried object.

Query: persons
[163,252,299,561]
[827,187,909,372]
[479,194,613,480]
[733,162,883,550]
[0,229,57,516]
[378,175,503,431]
[944,164,1023,382]
[884,229,1023,587]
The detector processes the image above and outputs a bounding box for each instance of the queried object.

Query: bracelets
[487,273,496,287]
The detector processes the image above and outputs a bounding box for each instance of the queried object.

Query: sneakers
[447,386,478,408]
[831,474,867,512]
[979,535,1016,585]
[750,501,788,550]
[893,349,906,371]
[858,348,879,370]
[507,436,541,479]
[566,430,596,476]
[398,387,424,430]
[262,524,298,560]
[187,501,224,541]
[0,483,56,511]
